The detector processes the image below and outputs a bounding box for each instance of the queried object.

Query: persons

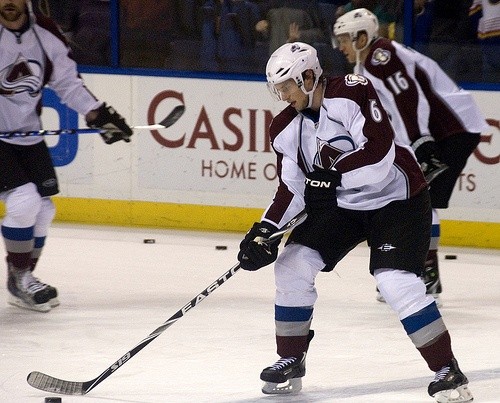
[0,0,132,313]
[238,43,475,402]
[332,8,488,305]
[32,0,500,85]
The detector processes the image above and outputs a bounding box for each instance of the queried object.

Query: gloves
[237,221,283,271]
[87,101,132,146]
[304,169,343,224]
[410,135,451,182]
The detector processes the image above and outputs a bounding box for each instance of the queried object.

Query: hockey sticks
[26,210,307,396]
[0,104,186,139]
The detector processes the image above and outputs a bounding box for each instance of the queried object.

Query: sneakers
[375,249,444,304]
[6,255,60,312]
[259,328,314,394]
[428,355,474,403]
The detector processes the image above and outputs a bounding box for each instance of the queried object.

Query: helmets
[329,8,381,55]
[265,41,323,97]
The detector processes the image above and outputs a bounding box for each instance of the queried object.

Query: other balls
[143,238,155,244]
[44,397,62,403]
[444,254,458,260]
[215,246,228,251]
